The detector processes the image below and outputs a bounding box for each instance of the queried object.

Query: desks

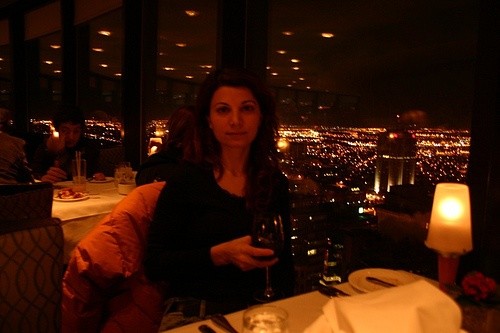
[50,171,137,266]
[161,269,461,333]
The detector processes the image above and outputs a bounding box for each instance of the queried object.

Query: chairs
[0,143,167,333]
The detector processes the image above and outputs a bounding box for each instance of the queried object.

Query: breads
[93,173,105,180]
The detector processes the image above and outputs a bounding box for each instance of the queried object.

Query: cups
[118,183,134,195]
[242,304,290,333]
[71,159,86,189]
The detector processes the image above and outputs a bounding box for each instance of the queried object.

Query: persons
[144,68,296,321]
[135,105,205,186]
[0,108,106,183]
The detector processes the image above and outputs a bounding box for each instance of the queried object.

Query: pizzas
[57,188,85,199]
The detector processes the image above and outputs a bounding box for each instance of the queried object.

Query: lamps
[425,183,474,287]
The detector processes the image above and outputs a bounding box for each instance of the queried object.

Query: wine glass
[252,213,285,303]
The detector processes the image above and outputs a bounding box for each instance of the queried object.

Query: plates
[53,192,90,202]
[348,267,414,293]
[88,176,113,183]
